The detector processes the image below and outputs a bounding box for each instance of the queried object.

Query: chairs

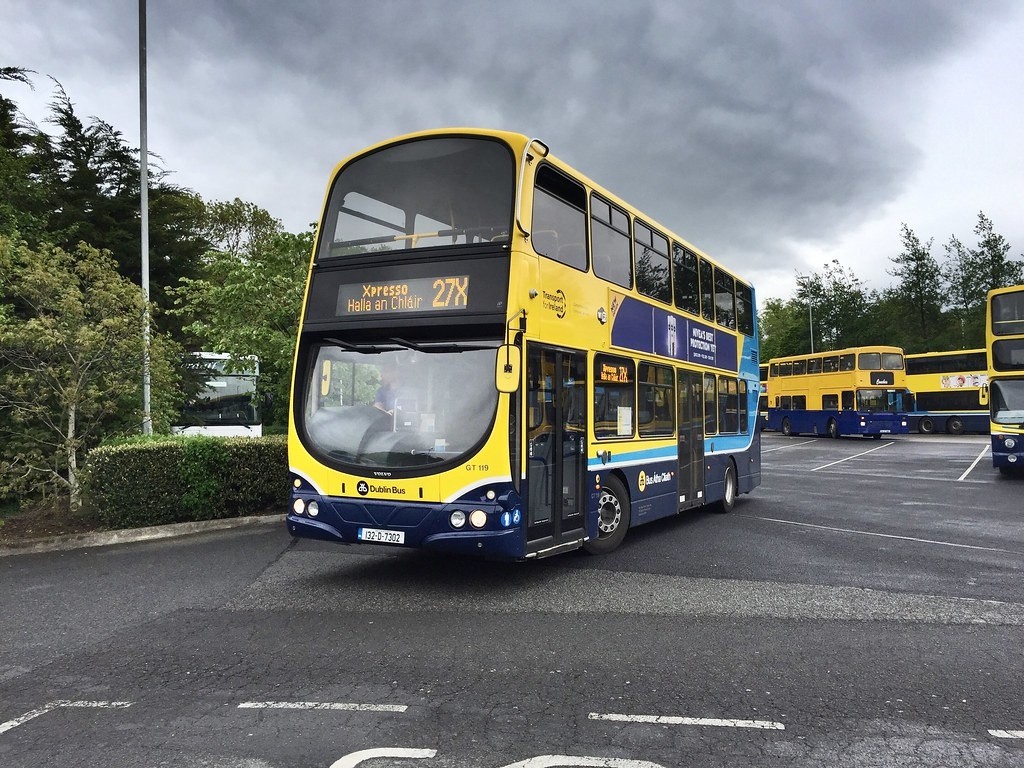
[442,231,746,436]
[790,362,887,414]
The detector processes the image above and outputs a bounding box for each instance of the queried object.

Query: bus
[978,284,1024,475]
[286,128,761,565]
[169,352,273,439]
[767,346,916,439]
[903,349,989,434]
[759,363,770,432]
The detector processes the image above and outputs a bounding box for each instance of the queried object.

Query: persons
[376,367,410,418]
